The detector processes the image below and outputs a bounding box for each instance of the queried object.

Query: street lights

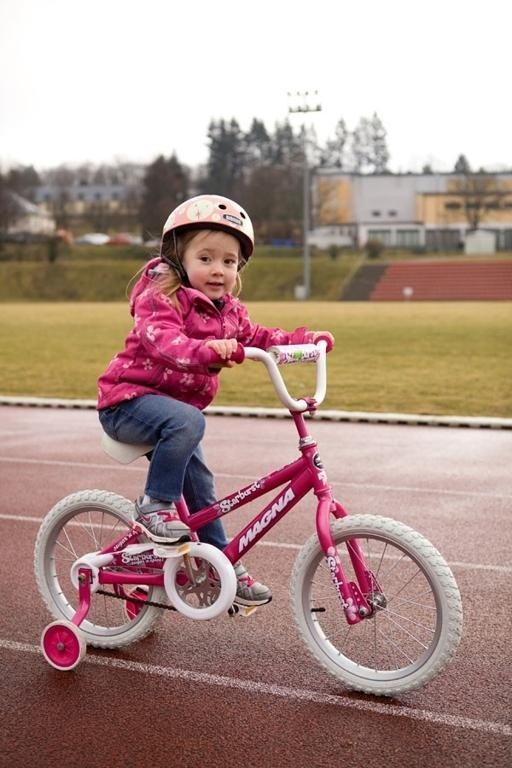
[285,88,323,295]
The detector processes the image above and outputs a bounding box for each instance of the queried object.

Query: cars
[75,232,134,246]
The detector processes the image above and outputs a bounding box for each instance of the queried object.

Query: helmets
[159,193,256,273]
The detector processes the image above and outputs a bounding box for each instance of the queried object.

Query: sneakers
[211,559,273,608]
[131,494,193,547]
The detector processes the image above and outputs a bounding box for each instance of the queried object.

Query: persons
[97,194,335,606]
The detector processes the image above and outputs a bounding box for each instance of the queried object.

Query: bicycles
[33,336,464,699]
